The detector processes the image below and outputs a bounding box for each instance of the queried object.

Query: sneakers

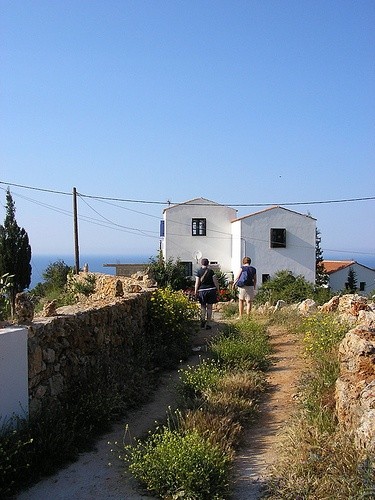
[201,319,206,328]
[206,325,211,329]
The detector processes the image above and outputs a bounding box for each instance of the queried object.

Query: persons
[194,258,220,329]
[232,257,257,321]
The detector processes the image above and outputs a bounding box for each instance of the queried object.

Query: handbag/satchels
[236,266,248,287]
